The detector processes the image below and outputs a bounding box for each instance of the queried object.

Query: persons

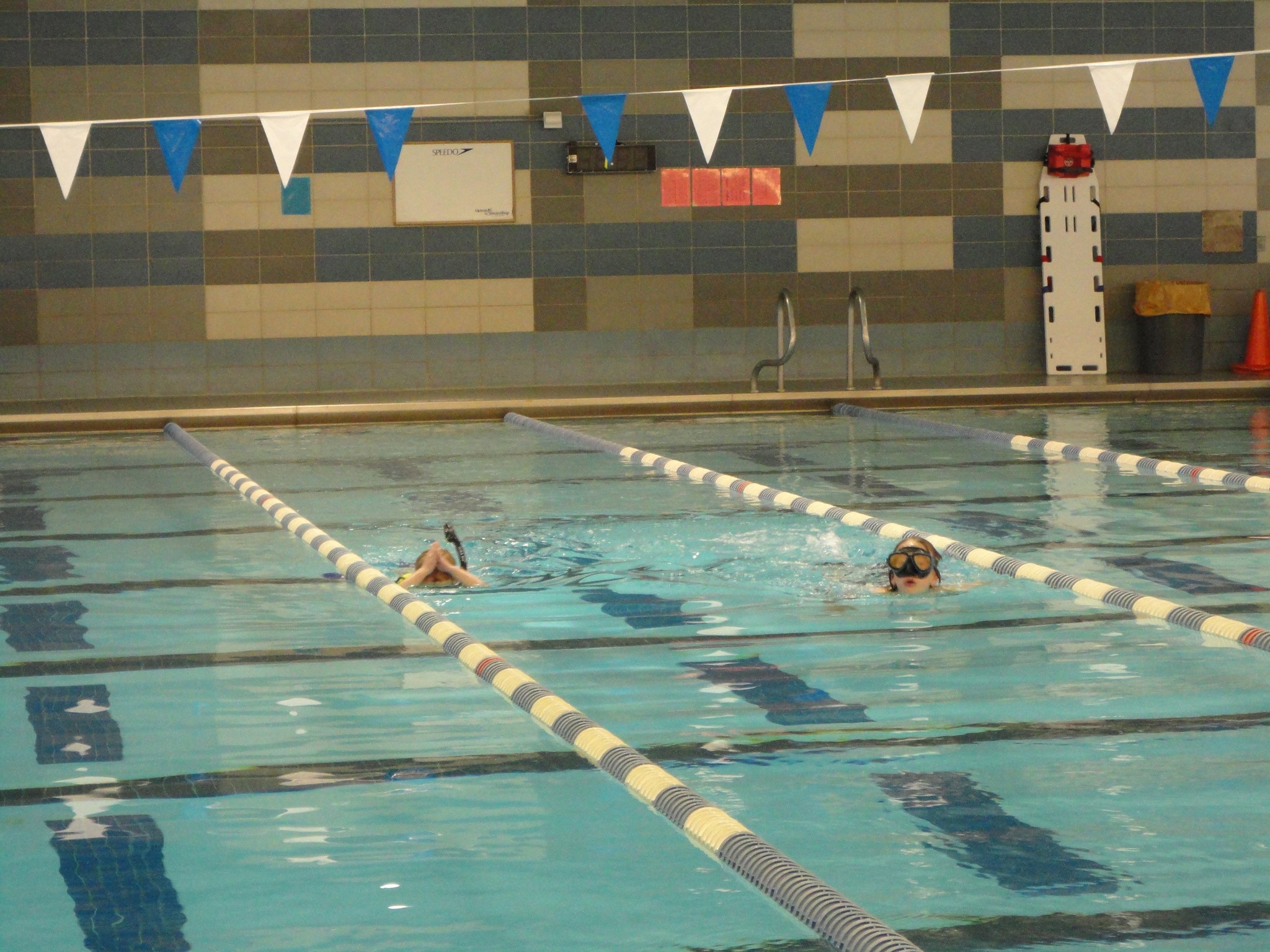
[843,536,1007,602]
[395,539,491,594]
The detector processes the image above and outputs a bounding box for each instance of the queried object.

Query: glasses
[887,547,937,579]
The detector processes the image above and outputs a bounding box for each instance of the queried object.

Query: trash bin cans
[1132,279,1211,377]
